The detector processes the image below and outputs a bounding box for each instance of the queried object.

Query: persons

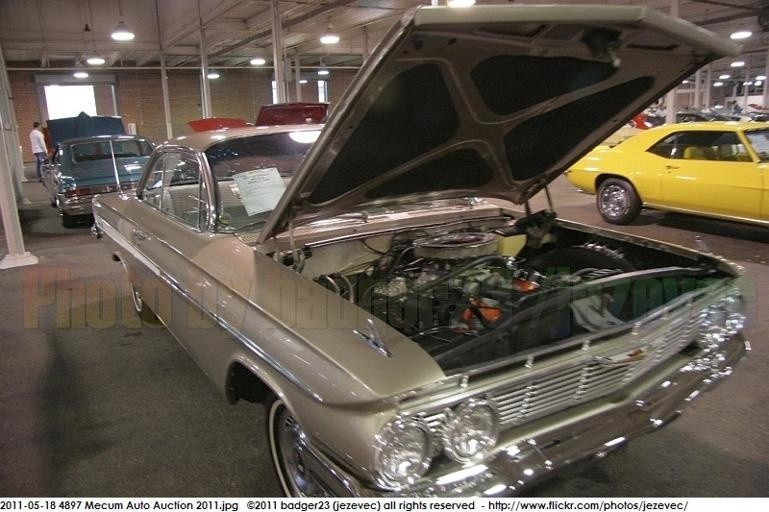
[28,121,49,183]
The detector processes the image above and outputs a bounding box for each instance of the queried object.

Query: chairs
[718,142,732,158]
[684,146,706,160]
[701,147,715,159]
[165,177,291,227]
